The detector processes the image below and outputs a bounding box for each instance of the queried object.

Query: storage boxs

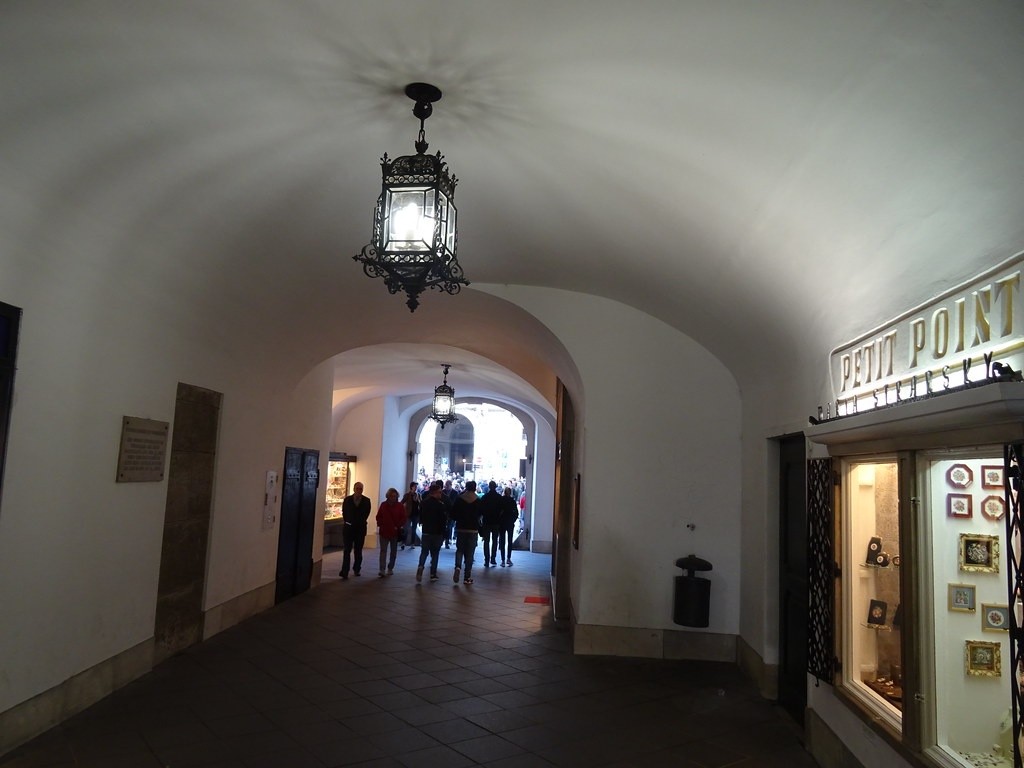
[891,663,901,685]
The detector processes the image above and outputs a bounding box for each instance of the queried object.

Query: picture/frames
[958,533,1000,575]
[981,495,1006,521]
[980,464,1005,489]
[948,583,977,613]
[947,493,973,519]
[964,639,1001,679]
[946,464,973,488]
[981,603,1009,633]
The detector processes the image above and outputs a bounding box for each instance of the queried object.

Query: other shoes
[445,545,450,549]
[401,544,405,549]
[500,563,505,566]
[453,567,460,583]
[490,561,497,564]
[387,568,393,575]
[484,563,489,567]
[430,575,439,582]
[416,567,422,582]
[378,570,385,577]
[463,577,475,584]
[339,572,348,578]
[507,560,513,565]
[411,544,415,549]
[354,571,360,576]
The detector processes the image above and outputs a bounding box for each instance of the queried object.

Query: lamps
[427,363,459,430]
[352,82,472,316]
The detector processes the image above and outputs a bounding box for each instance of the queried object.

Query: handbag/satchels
[398,527,406,542]
[479,520,487,537]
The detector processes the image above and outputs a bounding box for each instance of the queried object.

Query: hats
[410,481,418,489]
[489,481,498,489]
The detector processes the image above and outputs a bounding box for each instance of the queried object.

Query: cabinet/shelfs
[323,452,357,549]
[858,561,899,636]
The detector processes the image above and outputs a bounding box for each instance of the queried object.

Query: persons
[338,482,371,579]
[376,465,527,584]
